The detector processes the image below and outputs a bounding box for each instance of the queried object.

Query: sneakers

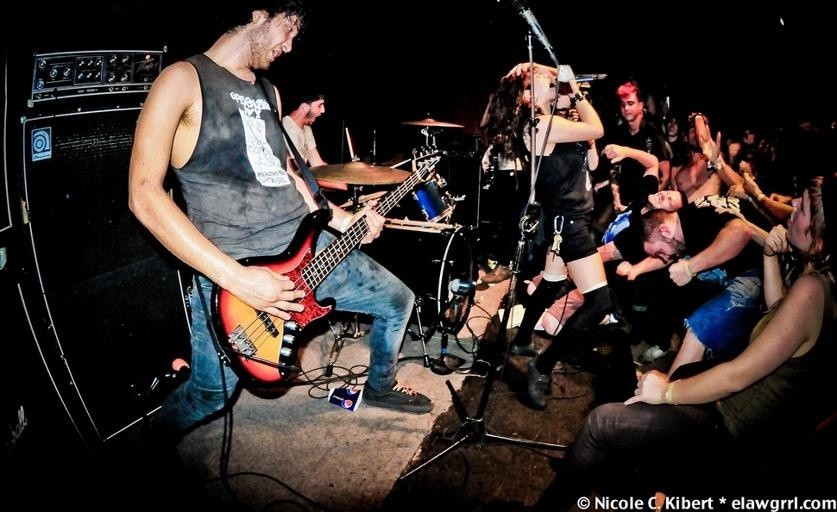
[362,381,433,414]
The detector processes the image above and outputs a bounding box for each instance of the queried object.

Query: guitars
[211,151,446,398]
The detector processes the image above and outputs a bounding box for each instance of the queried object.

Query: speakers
[13,102,193,446]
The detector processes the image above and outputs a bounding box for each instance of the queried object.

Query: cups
[328,386,365,413]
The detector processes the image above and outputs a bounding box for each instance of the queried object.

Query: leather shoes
[527,360,550,408]
[510,341,548,355]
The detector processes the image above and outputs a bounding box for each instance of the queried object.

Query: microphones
[515,1,553,56]
[171,356,192,381]
[554,70,609,88]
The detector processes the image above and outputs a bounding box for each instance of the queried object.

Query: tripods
[311,226,571,491]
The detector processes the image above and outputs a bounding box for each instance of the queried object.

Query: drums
[369,217,475,334]
[380,178,452,222]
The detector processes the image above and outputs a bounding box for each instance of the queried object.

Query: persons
[127,2,434,468]
[283,92,348,191]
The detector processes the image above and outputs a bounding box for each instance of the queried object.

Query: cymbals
[310,162,411,186]
[403,117,464,130]
